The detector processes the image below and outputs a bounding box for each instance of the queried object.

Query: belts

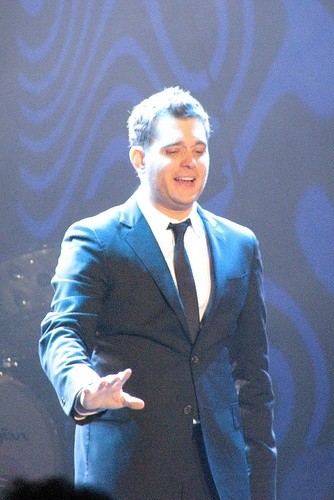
[192,423,204,439]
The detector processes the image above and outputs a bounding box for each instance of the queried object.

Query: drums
[0,360,74,500]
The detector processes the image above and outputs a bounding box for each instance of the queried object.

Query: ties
[167,218,201,344]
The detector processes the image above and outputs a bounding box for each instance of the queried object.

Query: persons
[39,87,278,500]
[0,475,113,500]
